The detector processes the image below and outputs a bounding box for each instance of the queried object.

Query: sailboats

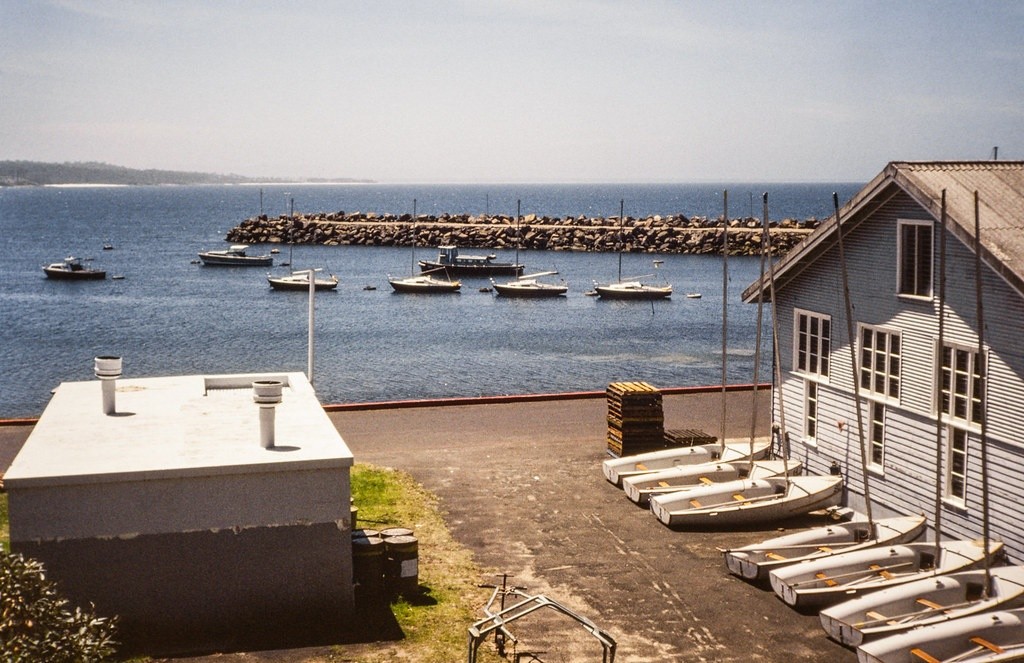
[493,198,569,298]
[768,186,1008,612]
[820,187,1024,652]
[386,197,464,292]
[718,189,927,583]
[593,197,673,299]
[42,256,107,279]
[599,189,845,529]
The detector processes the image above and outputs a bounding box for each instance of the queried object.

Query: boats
[197,244,273,266]
[854,607,1024,663]
[417,245,525,276]
[265,198,339,290]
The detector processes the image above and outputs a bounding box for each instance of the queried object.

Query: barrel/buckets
[350,527,419,598]
